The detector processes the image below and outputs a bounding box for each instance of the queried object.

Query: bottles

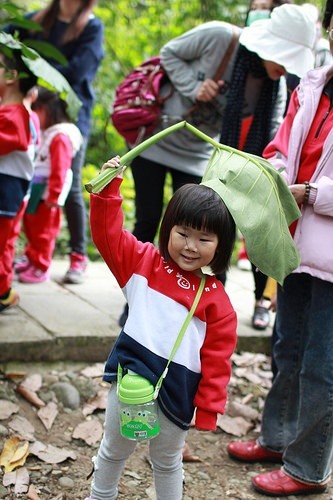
[117,368,161,442]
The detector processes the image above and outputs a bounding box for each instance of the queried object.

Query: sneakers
[63,253,89,282]
[0,288,19,312]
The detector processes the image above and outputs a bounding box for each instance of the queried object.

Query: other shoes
[253,468,327,495]
[19,267,46,283]
[253,306,270,330]
[14,261,29,273]
[227,439,283,464]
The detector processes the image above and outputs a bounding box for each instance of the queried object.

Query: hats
[246,9,270,26]
[238,3,316,79]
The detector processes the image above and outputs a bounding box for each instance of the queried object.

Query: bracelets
[303,182,310,205]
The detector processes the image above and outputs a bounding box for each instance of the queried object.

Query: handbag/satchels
[112,56,167,145]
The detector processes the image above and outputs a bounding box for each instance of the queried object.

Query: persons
[16,86,83,283]
[242,0,333,329]
[228,0,333,496]
[0,53,37,314]
[86,154,238,500]
[118,4,317,327]
[0,0,104,284]
